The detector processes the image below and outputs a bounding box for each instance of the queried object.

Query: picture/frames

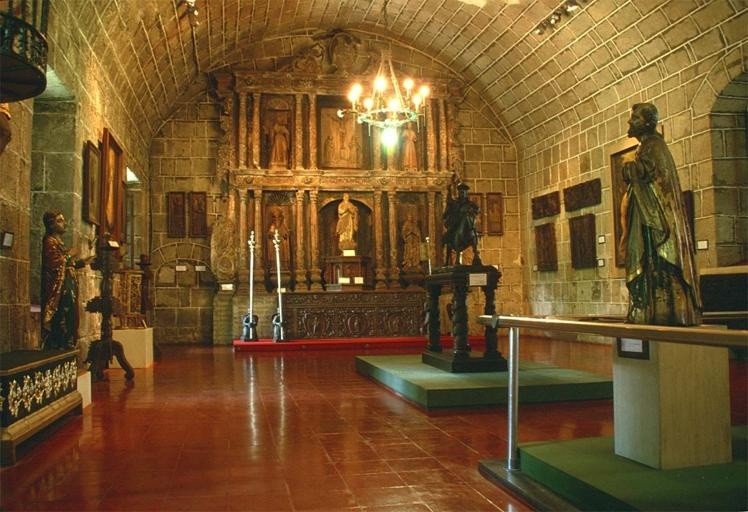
[190,191,207,238]
[682,190,695,254]
[534,223,558,271]
[532,191,560,219]
[85,140,103,227]
[569,214,596,268]
[469,193,483,235]
[100,127,123,250]
[564,179,601,211]
[168,191,186,238]
[486,193,503,235]
[610,143,640,267]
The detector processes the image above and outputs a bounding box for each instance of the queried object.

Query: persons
[401,212,422,267]
[348,136,361,163]
[40,208,97,351]
[438,184,481,248]
[622,103,697,326]
[268,114,290,164]
[400,121,417,171]
[335,194,358,250]
[324,135,335,165]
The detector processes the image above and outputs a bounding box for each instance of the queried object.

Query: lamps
[347,0,429,150]
